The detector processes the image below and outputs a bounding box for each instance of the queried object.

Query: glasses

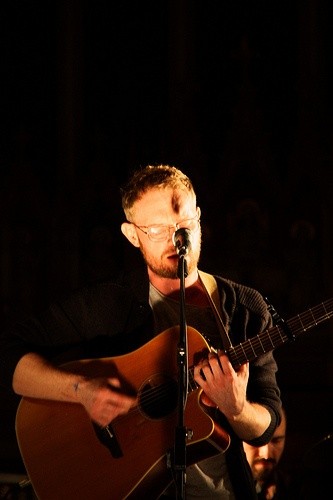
[131,214,200,242]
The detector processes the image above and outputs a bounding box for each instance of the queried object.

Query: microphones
[172,227,191,257]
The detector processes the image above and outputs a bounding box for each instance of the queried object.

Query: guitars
[13,290,332,498]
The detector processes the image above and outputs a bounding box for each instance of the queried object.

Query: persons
[6,161,281,500]
[241,405,287,500]
